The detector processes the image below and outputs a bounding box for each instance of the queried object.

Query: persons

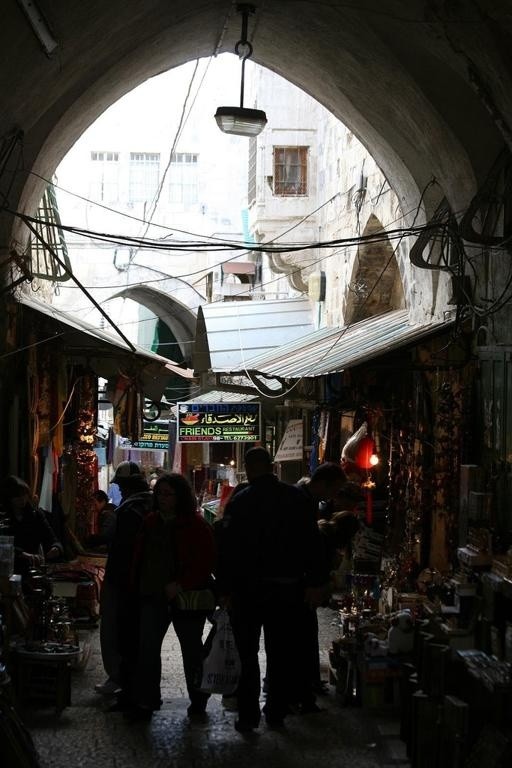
[90,442,357,732]
[0,473,64,577]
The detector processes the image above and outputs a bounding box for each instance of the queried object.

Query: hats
[109,461,144,482]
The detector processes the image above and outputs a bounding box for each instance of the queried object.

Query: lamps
[369,432,379,465]
